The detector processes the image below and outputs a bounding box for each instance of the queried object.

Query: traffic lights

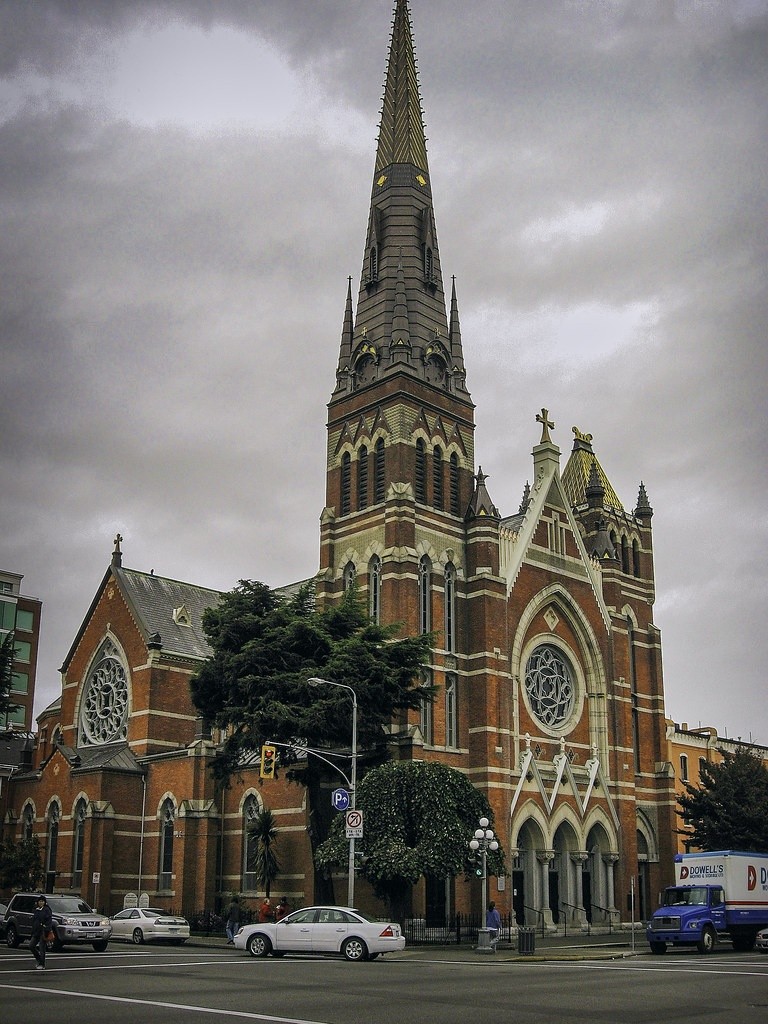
[260,745,275,778]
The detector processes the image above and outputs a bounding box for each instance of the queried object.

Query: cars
[754,928,768,954]
[233,906,405,963]
[0,903,8,941]
[109,907,190,945]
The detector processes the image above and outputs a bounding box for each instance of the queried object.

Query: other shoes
[226,940,233,945]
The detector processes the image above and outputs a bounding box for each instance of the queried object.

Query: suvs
[3,893,113,953]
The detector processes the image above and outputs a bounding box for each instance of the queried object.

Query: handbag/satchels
[42,930,56,942]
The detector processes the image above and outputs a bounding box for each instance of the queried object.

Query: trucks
[645,850,768,956]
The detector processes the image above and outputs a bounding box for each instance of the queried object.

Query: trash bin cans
[517,926,536,956]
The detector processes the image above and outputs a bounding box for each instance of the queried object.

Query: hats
[37,895,46,903]
[280,895,288,904]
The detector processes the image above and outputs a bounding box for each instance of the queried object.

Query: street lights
[307,676,357,909]
[469,818,499,955]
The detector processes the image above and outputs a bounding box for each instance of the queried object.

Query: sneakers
[35,963,46,970]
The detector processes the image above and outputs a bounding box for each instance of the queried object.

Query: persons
[28,895,52,970]
[259,898,275,923]
[225,896,242,945]
[275,896,291,920]
[486,901,503,953]
